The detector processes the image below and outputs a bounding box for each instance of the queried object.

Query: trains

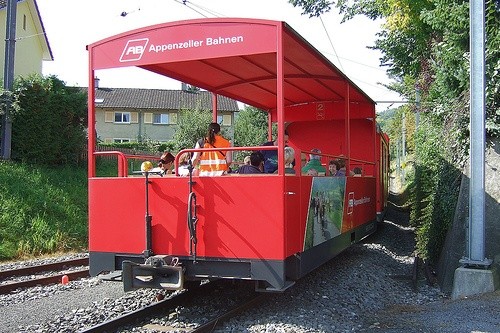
[86,16,390,296]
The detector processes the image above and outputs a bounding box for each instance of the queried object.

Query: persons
[354,167,361,177]
[142,161,153,176]
[232,152,263,174]
[179,152,191,176]
[192,123,232,176]
[329,155,352,176]
[302,149,326,174]
[274,147,295,174]
[244,156,251,165]
[260,130,289,173]
[153,152,174,174]
